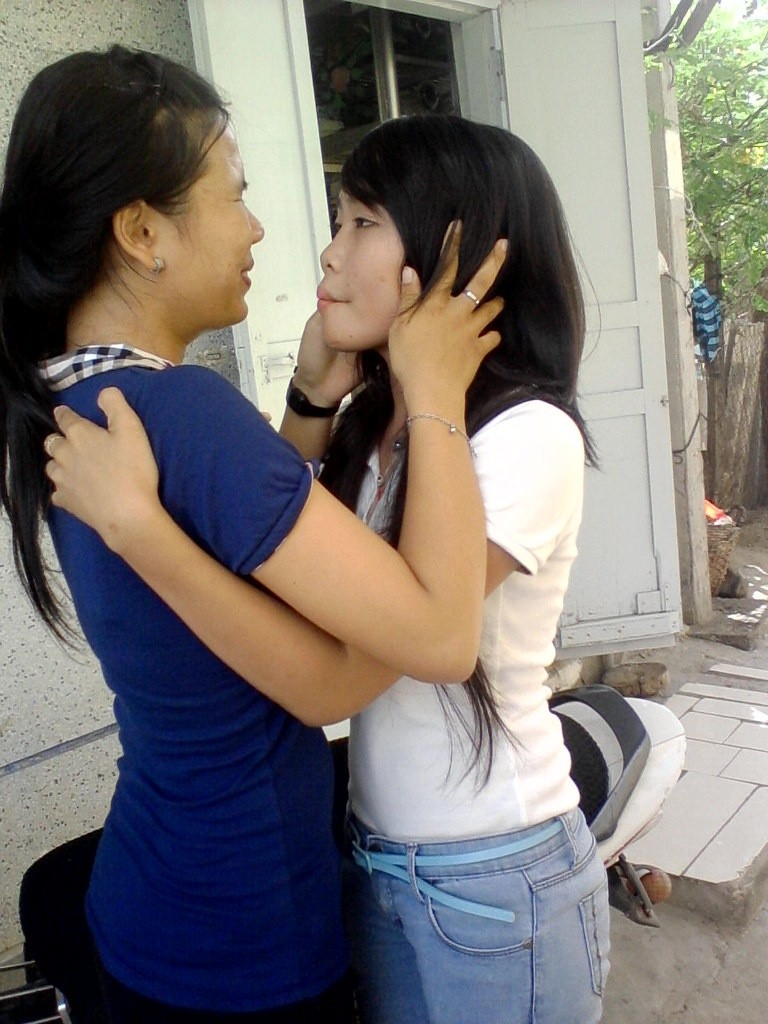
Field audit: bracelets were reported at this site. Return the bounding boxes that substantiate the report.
[404,411,479,463]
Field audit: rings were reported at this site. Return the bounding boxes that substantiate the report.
[465,286,480,307]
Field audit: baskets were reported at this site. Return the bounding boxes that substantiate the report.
[707,505,745,596]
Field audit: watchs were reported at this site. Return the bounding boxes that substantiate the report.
[284,365,341,418]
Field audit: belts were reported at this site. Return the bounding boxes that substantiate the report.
[352,822,564,922]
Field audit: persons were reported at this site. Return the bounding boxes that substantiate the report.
[44,111,616,1024]
[1,40,513,1024]
